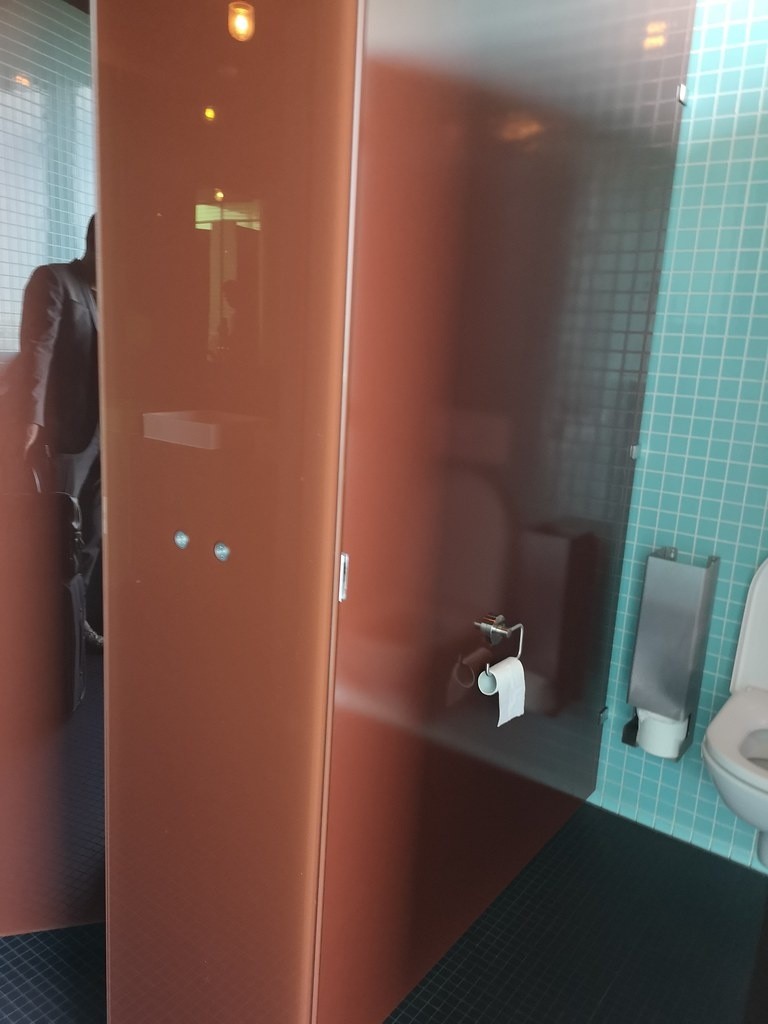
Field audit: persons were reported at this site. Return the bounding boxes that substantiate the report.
[14,212,105,716]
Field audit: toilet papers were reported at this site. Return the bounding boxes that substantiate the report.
[635,708,689,760]
[477,656,526,728]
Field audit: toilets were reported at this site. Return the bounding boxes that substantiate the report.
[701,556,767,877]
